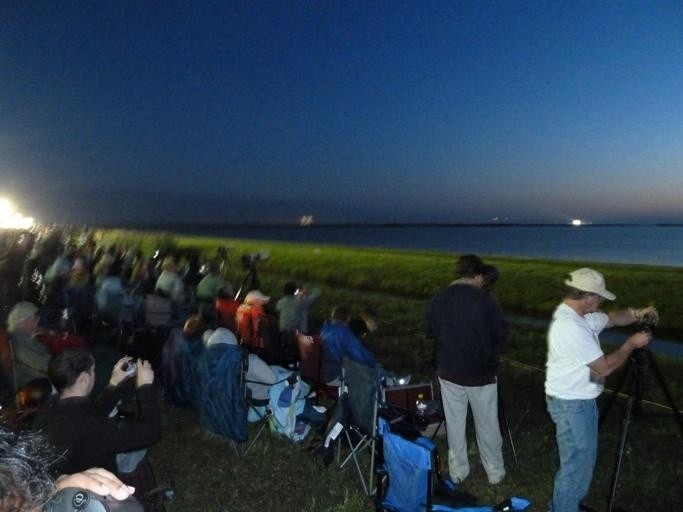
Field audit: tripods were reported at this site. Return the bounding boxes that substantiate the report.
[598,346,682,512]
[232,272,263,301]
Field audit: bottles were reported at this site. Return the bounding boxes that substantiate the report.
[416,394,427,417]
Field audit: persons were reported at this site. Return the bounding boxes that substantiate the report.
[1,224,383,512]
[424,254,514,486]
[544,267,660,511]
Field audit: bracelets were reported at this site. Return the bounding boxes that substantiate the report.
[630,308,640,323]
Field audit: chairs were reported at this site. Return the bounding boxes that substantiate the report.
[238,355,316,456]
[336,355,434,498]
[376,432,493,512]
[0,338,48,407]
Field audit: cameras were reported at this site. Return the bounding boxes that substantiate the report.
[123,361,139,374]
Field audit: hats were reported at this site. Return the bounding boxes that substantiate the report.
[244,291,271,305]
[563,268,616,300]
